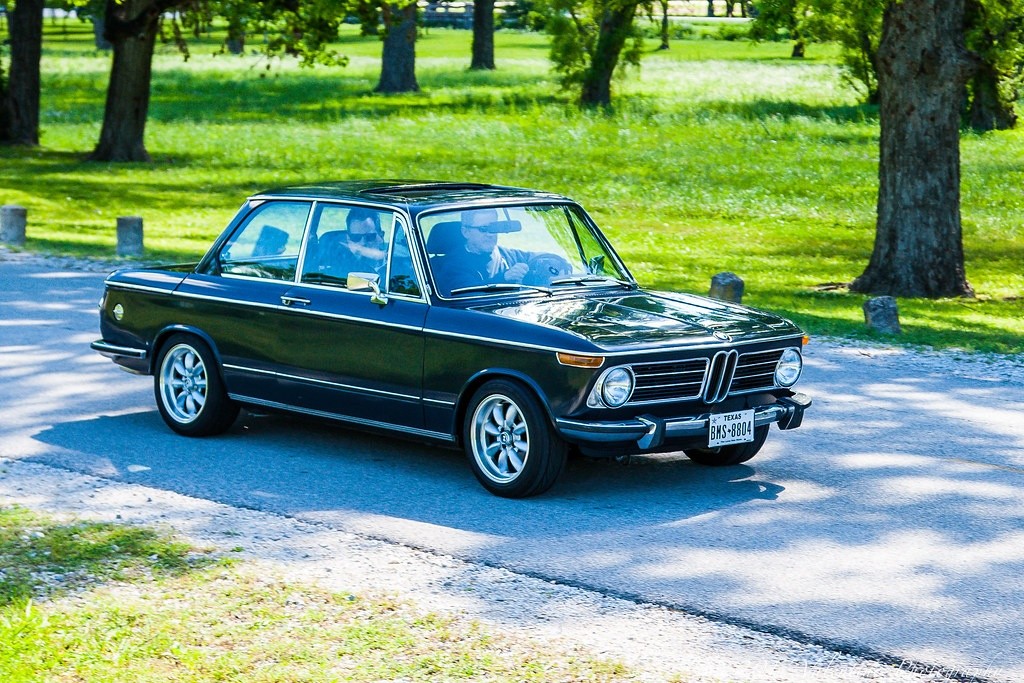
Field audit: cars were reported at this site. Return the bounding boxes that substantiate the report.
[89,177,813,501]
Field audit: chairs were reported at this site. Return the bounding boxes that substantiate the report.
[318,230,350,273]
[427,221,463,272]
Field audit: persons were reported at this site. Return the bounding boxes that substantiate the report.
[439,208,572,294]
[329,208,416,295]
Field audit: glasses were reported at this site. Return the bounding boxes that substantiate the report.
[347,231,379,243]
[465,224,488,233]
